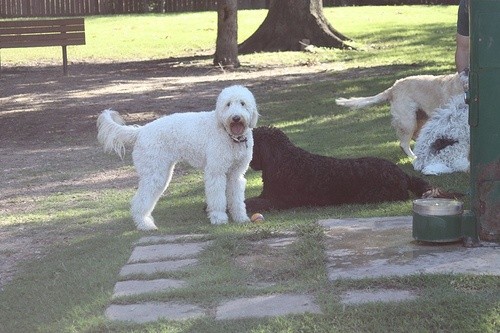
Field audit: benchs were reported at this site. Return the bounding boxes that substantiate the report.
[0,18,86,76]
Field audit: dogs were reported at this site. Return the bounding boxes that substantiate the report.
[334,66,469,158]
[203,125,468,213]
[96,84,260,232]
[408,93,470,176]
